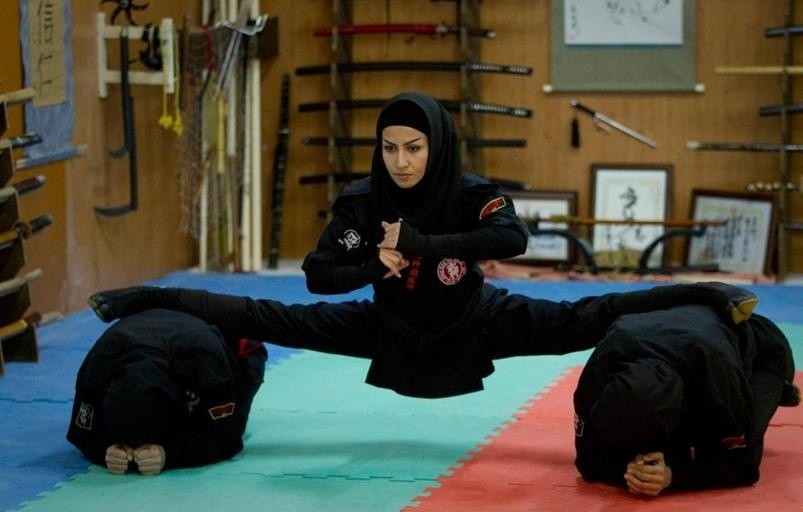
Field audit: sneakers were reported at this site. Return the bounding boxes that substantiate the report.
[87,286,147,324]
[710,282,758,325]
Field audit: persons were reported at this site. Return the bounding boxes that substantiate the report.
[65,308,269,477]
[570,302,801,498]
[87,90,759,398]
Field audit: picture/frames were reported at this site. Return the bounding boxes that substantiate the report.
[497,161,778,278]
